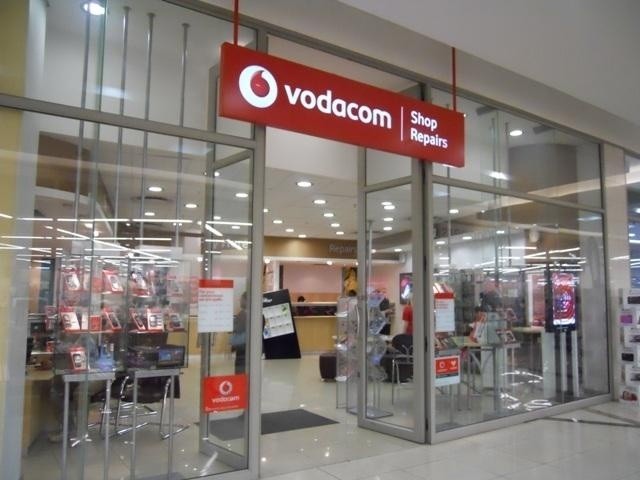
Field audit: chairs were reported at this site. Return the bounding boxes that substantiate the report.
[391,333,414,405]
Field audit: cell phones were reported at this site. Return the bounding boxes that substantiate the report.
[135,315,143,327]
[109,314,118,327]
[73,354,82,367]
[169,280,179,292]
[110,277,120,289]
[150,315,157,327]
[171,315,180,327]
[135,272,144,287]
[66,275,77,288]
[63,314,72,328]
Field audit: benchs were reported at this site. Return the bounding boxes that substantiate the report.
[319,352,413,384]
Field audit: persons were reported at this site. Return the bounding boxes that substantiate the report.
[344,268,357,295]
[401,292,413,336]
[297,297,305,302]
[230,288,265,420]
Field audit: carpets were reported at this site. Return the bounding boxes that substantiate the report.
[194,408,340,442]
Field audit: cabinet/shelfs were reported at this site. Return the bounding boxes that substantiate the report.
[21,253,190,457]
[335,293,393,422]
